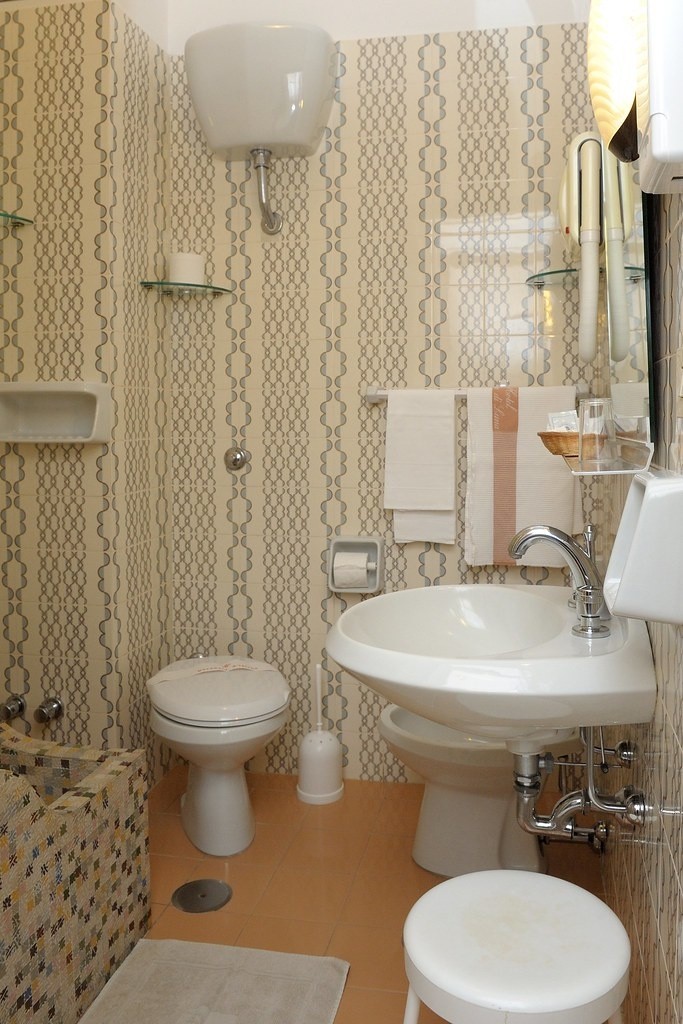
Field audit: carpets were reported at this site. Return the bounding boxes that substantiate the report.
[77,938,350,1024]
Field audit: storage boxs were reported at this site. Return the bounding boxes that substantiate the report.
[537,430,609,457]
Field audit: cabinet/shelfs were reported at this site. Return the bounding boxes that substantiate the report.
[1,379,113,448]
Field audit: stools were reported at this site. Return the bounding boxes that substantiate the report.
[400,866,631,1024]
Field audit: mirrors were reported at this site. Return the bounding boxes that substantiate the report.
[600,141,653,446]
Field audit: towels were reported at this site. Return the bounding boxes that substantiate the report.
[383,383,584,568]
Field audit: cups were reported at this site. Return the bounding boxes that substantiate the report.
[577,398,619,463]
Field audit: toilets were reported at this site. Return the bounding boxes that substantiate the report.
[147,655,292,859]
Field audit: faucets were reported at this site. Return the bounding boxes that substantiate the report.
[507,525,615,621]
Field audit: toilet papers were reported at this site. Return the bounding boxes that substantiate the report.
[165,252,205,288]
[332,551,371,590]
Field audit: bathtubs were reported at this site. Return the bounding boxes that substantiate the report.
[0,719,153,1024]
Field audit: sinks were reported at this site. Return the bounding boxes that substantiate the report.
[325,582,659,757]
[377,703,584,881]
